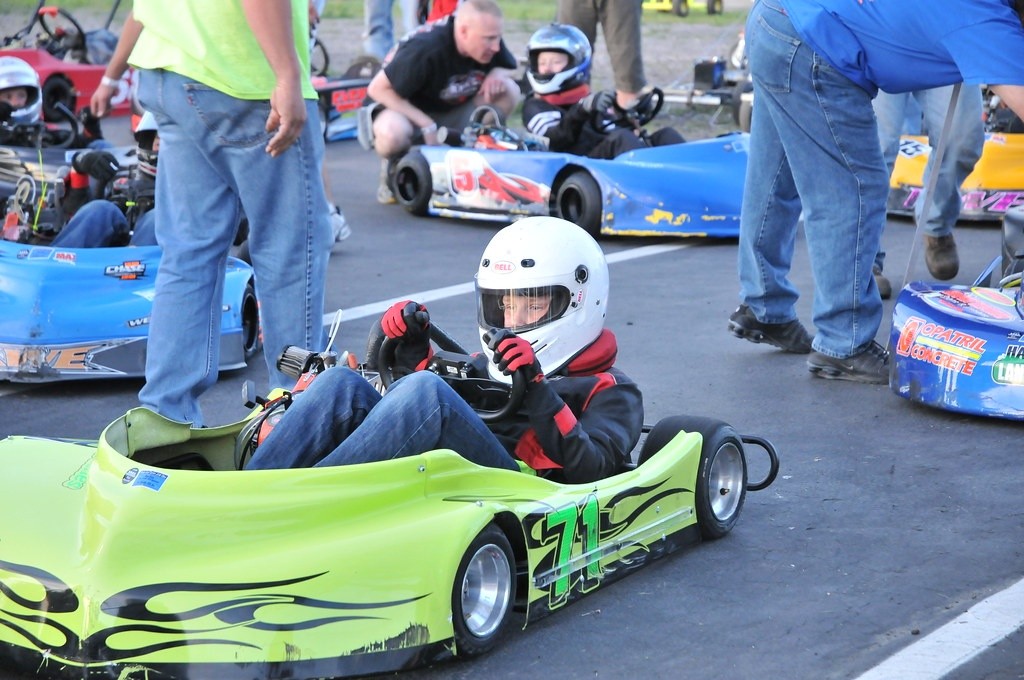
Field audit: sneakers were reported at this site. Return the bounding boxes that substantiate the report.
[925,233,958,281]
[375,186,397,204]
[728,304,814,353]
[806,341,891,386]
[328,203,351,243]
[870,268,892,298]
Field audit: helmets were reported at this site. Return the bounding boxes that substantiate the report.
[521,24,592,95]
[134,111,158,176]
[0,56,42,126]
[475,216,609,386]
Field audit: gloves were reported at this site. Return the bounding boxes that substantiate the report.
[488,330,548,385]
[576,90,616,116]
[380,300,430,353]
[0,101,17,125]
[72,152,119,180]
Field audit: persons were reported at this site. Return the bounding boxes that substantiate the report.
[118,0,337,429]
[245,216,644,485]
[729,0,1024,385]
[0,0,985,297]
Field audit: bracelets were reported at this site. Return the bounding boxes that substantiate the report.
[421,123,438,133]
[101,77,120,89]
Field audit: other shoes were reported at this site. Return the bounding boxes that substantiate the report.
[624,93,653,120]
